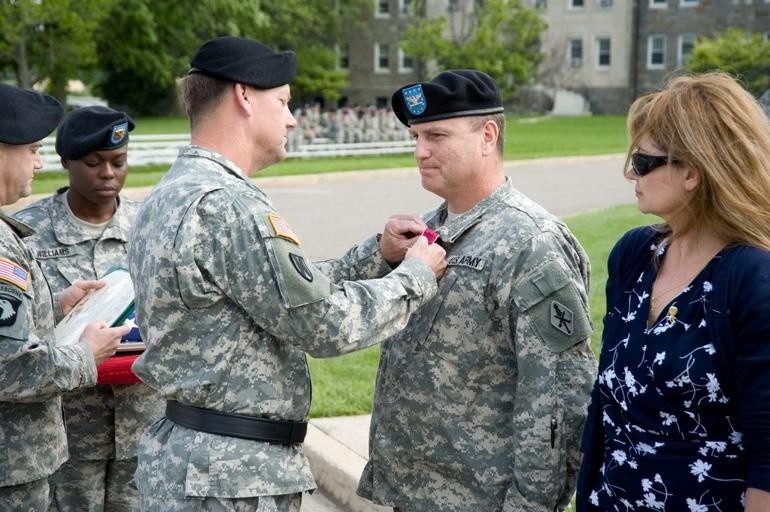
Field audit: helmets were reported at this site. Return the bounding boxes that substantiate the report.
[1,80,64,146]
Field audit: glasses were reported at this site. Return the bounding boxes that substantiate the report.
[629,149,684,178]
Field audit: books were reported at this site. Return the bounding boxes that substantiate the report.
[53,265,137,348]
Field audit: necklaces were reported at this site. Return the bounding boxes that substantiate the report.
[645,259,696,316]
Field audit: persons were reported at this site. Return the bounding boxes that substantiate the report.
[352,69,597,511]
[8,105,148,511]
[572,66,769,510]
[127,35,449,512]
[0,79,135,509]
[285,101,413,154]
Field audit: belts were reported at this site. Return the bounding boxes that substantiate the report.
[158,397,309,449]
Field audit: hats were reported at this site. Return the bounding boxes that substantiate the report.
[185,35,300,88]
[391,67,505,129]
[55,105,136,159]
